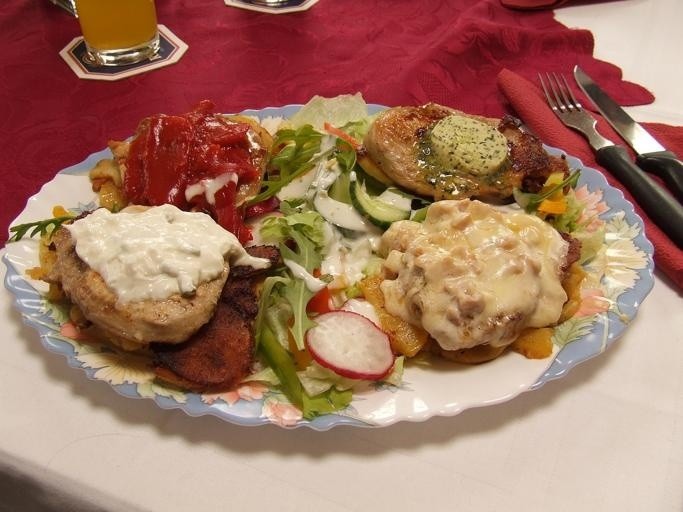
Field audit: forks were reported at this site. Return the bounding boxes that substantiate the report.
[535,71,681,256]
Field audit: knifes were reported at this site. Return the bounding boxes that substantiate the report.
[573,62,682,209]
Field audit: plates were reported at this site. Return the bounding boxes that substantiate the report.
[1,103,656,435]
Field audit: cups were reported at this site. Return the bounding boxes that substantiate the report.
[72,0,163,66]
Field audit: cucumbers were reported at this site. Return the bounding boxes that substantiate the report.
[349,178,413,231]
